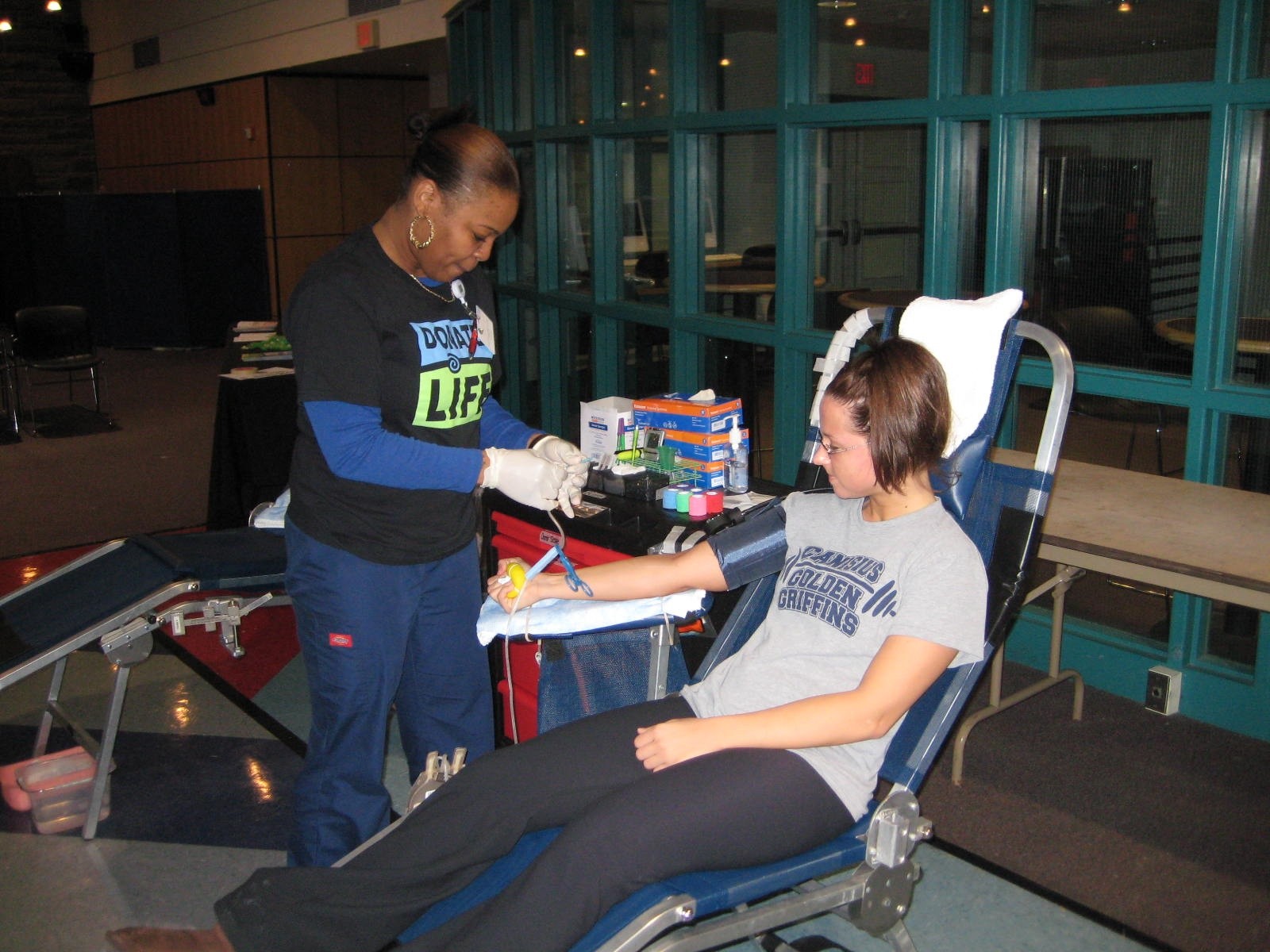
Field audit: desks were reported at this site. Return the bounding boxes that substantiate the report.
[206,322,299,533]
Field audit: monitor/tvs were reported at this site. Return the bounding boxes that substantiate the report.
[624,200,650,258]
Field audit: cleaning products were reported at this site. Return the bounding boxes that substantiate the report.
[722,413,749,494]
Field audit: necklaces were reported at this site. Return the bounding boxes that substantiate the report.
[410,274,456,302]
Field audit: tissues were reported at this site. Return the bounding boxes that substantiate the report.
[632,388,744,434]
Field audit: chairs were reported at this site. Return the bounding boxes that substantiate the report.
[4,305,106,441]
[329,288,1074,952]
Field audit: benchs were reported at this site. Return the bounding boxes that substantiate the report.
[952,449,1270,785]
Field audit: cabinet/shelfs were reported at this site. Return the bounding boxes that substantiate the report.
[491,479,792,746]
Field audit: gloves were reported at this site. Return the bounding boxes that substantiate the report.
[480,446,566,512]
[533,434,589,520]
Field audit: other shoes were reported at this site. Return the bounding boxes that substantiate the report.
[106,921,237,952]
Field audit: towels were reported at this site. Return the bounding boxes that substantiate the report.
[898,285,1025,459]
[475,588,708,649]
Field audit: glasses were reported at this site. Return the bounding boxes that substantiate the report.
[815,437,872,455]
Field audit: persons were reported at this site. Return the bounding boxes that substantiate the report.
[103,338,989,952]
[284,119,593,870]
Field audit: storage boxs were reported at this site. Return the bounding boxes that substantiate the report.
[16,752,116,834]
[580,389,749,501]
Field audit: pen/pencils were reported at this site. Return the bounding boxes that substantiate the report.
[632,425,639,466]
[617,418,625,450]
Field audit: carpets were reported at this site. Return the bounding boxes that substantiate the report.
[916,662,1270,952]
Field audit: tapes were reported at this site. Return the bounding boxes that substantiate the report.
[231,367,258,375]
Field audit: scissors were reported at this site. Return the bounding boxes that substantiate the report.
[554,544,592,597]
